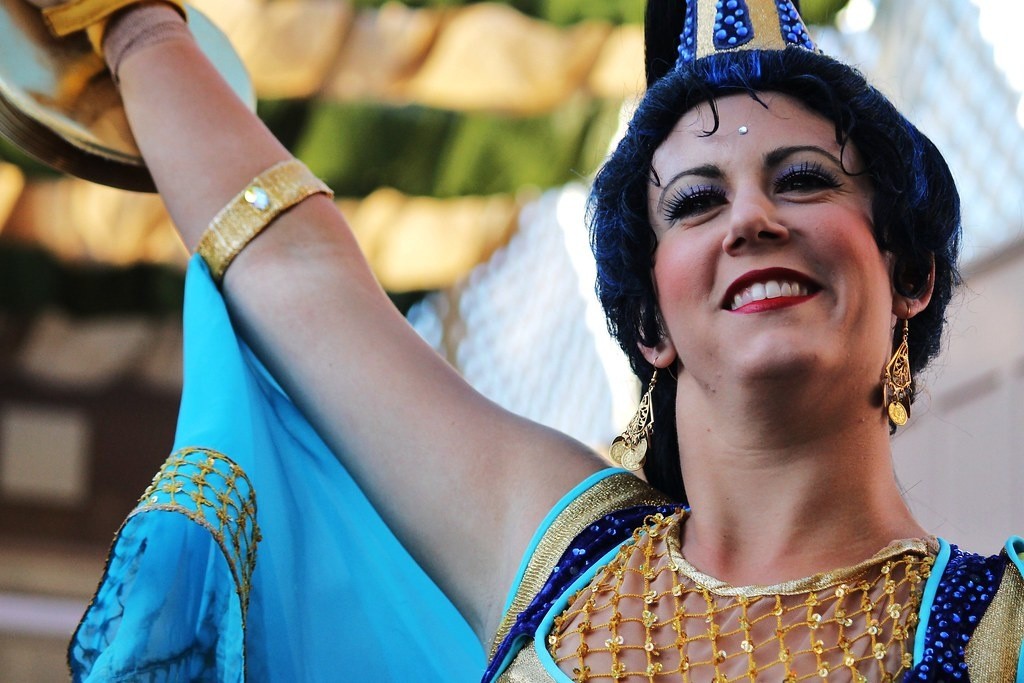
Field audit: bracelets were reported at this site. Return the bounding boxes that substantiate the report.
[98,7,194,84]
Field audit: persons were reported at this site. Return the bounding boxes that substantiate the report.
[26,0,1024,682]
